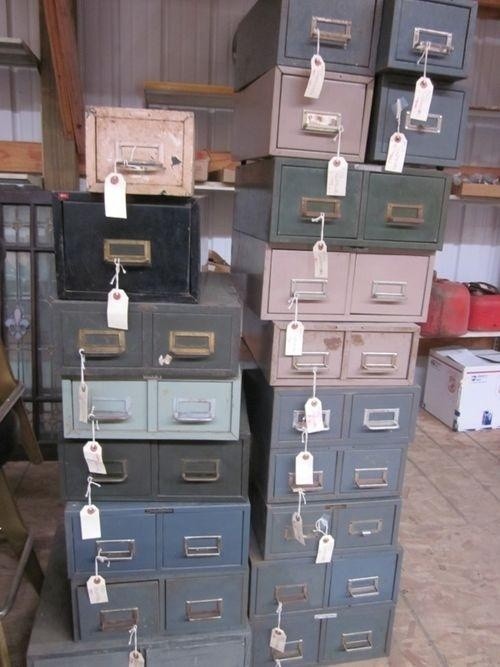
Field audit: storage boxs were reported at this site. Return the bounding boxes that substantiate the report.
[85,104,194,197]
[25,271,421,661]
[52,189,200,302]
[230,5,477,322]
[419,344,500,433]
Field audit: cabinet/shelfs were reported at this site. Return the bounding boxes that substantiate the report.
[140,79,236,193]
